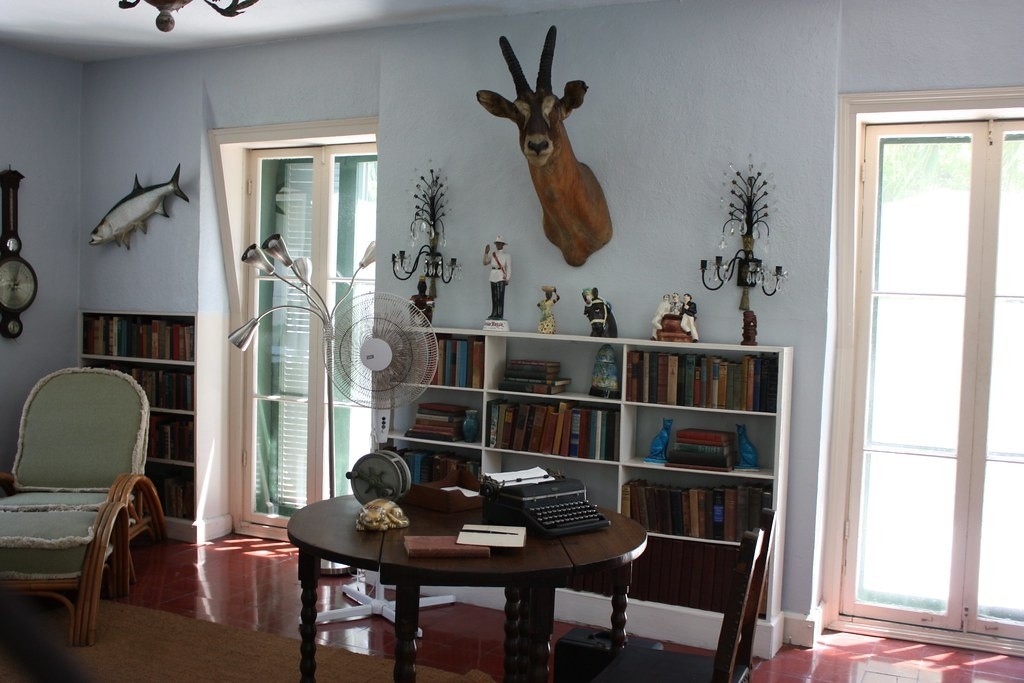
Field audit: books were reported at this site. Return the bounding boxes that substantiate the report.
[497,360,572,395]
[621,480,772,542]
[382,446,481,486]
[151,475,194,519]
[485,398,620,461]
[625,347,779,413]
[404,536,490,558]
[147,414,194,462]
[399,333,483,388]
[404,403,468,442]
[84,314,195,361]
[108,364,194,410]
[562,536,741,612]
[665,428,738,471]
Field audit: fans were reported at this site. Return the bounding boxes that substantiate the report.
[296,285,459,637]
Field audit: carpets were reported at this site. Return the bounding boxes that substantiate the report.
[30,598,496,683]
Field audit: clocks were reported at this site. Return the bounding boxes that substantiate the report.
[0,161,39,340]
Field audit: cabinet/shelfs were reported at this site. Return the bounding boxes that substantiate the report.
[372,320,796,663]
[75,310,198,539]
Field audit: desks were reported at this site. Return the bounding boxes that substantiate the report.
[284,479,650,683]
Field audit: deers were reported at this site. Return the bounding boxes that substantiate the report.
[475,23,615,269]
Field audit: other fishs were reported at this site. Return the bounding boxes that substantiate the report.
[88,162,190,251]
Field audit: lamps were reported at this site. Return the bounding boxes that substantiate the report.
[696,137,791,312]
[227,232,375,578]
[388,152,467,302]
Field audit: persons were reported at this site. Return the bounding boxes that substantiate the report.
[537,286,560,333]
[407,276,437,328]
[483,235,512,320]
[648,293,700,342]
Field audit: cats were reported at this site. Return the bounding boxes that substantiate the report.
[355,498,411,532]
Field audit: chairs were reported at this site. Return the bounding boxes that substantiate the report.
[0,365,170,649]
[580,507,780,683]
